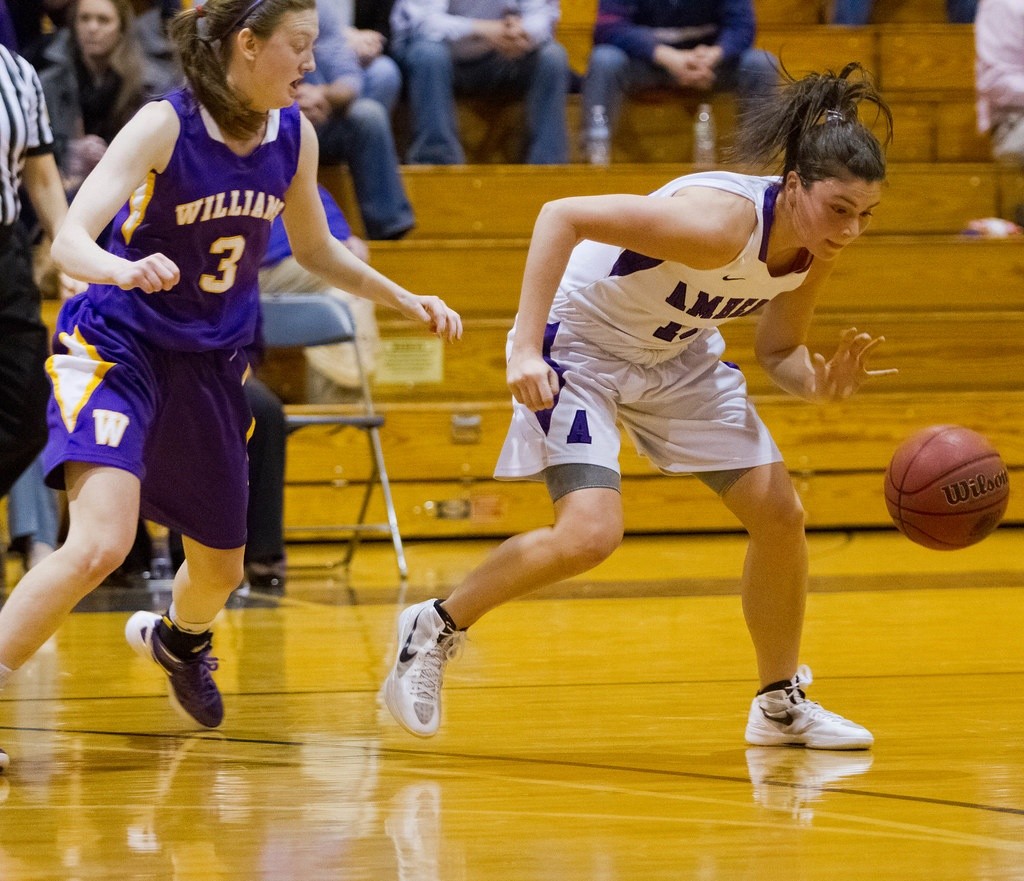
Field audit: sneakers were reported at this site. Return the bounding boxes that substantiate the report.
[0,749,11,776]
[746,665,874,750]
[125,609,225,727]
[387,599,476,739]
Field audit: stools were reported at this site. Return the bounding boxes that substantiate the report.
[256,294,409,581]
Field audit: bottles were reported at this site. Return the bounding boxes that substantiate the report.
[584,106,611,165]
[692,104,718,162]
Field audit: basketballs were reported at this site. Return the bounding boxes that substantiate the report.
[881,426,1011,551]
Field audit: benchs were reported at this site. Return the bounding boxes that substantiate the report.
[451,64,712,163]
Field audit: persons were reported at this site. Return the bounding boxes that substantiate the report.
[36,0,415,241]
[0,44,70,497]
[579,0,779,165]
[388,0,569,165]
[972,0,1024,156]
[386,59,893,750]
[0,0,462,774]
